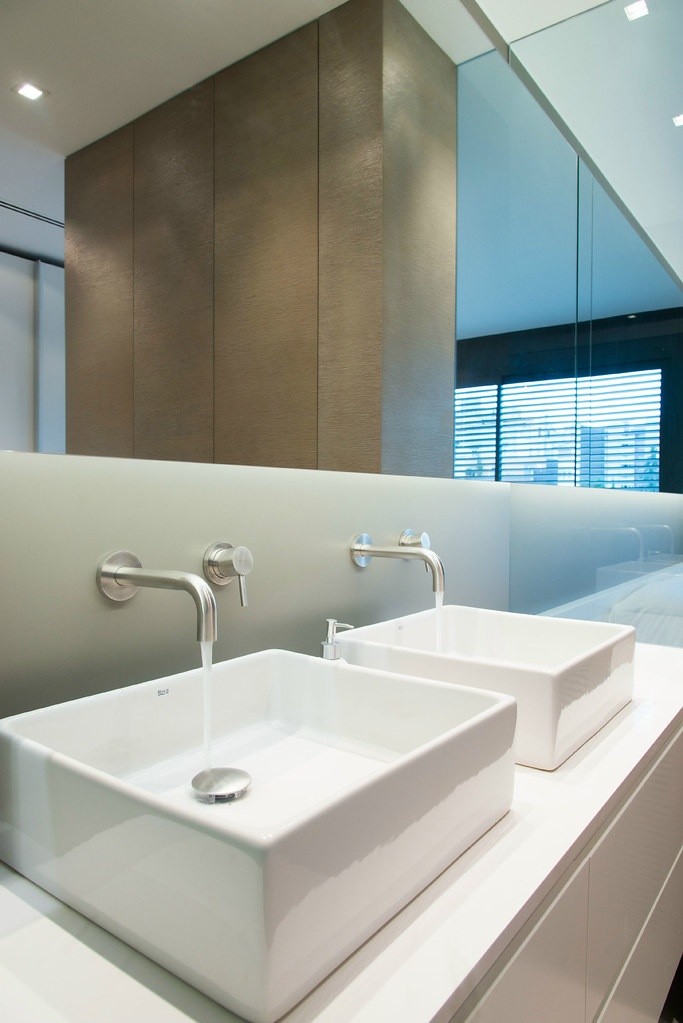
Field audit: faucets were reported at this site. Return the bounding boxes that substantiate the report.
[350,531,447,593]
[644,549,662,562]
[96,551,218,642]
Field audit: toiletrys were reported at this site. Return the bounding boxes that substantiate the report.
[320,619,354,664]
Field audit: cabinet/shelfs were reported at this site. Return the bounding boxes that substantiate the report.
[454,723,683,1023]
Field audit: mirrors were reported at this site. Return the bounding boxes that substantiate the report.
[0,0,683,500]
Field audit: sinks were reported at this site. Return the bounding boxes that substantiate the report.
[1,649,517,1023]
[325,604,636,771]
[595,552,683,617]
